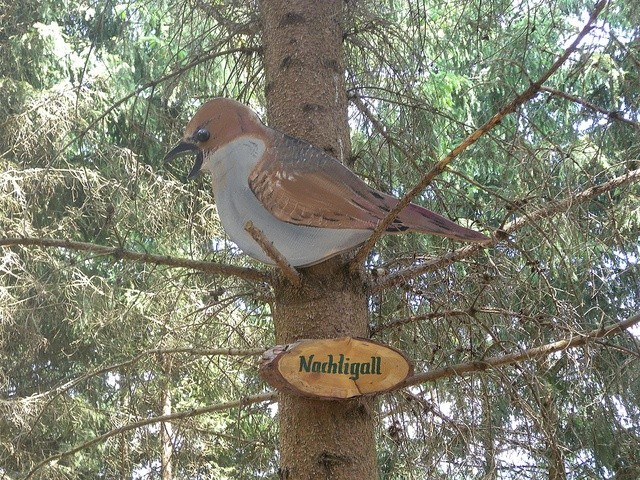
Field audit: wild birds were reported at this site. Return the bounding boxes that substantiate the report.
[164,97,491,269]
